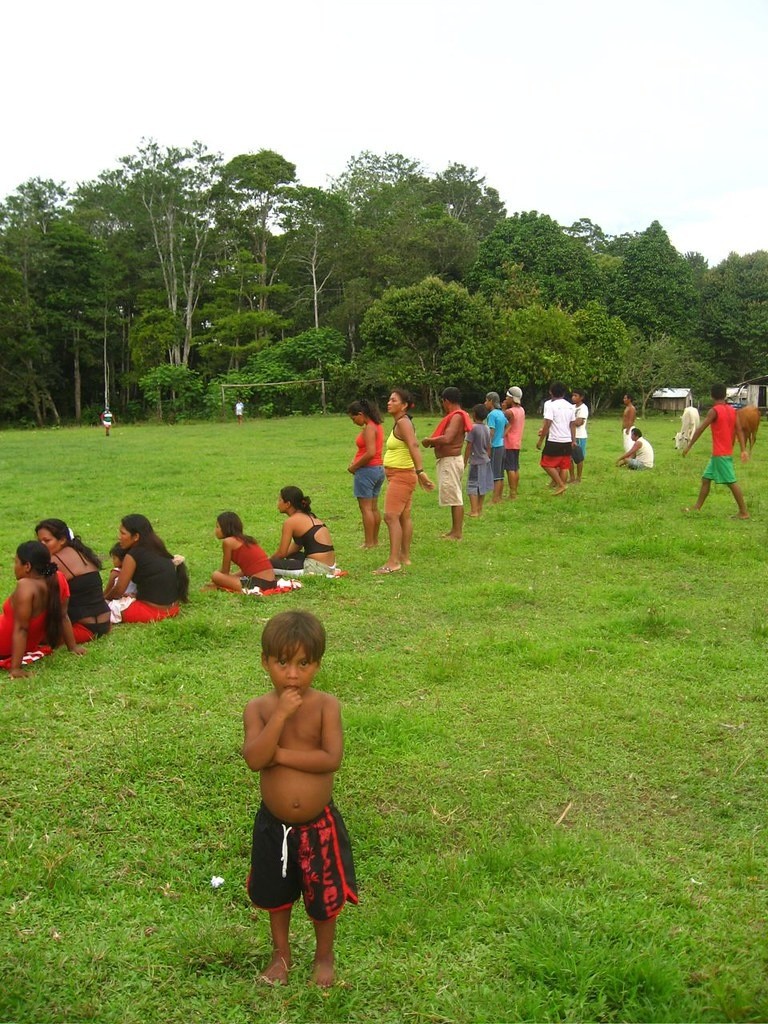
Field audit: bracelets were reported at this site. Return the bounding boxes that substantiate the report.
[416,469,423,474]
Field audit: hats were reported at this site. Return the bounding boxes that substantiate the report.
[487,392,502,409]
[506,386,523,404]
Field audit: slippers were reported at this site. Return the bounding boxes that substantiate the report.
[371,566,402,576]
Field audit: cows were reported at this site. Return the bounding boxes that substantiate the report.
[735,404,759,458]
[672,406,701,448]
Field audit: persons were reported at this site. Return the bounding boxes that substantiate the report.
[615,428,654,471]
[464,404,494,516]
[104,514,179,624]
[422,387,474,541]
[537,382,577,496]
[347,398,384,550]
[269,485,335,575]
[236,400,244,424]
[204,512,276,593]
[622,394,636,466]
[567,387,589,484]
[0,541,87,680]
[35,519,111,644]
[681,384,752,520]
[503,385,526,501]
[377,389,436,575]
[101,407,115,436]
[242,610,361,988]
[485,391,512,506]
